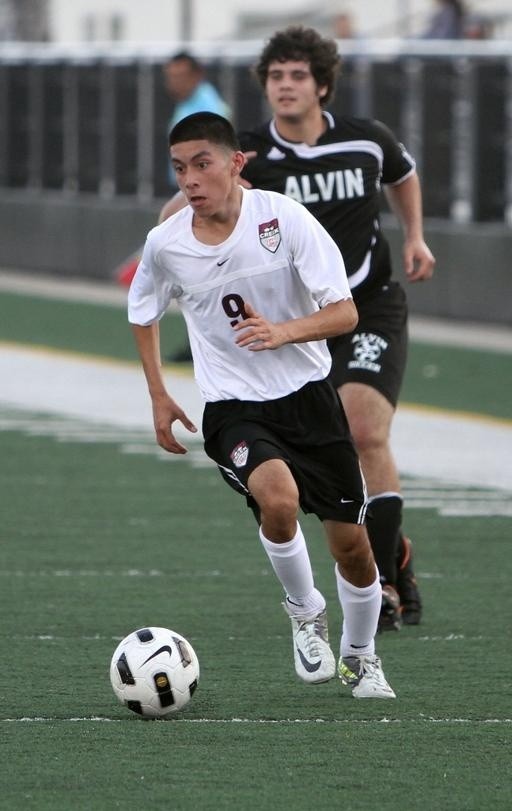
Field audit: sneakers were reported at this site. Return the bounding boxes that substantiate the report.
[337,655,397,698]
[378,535,421,632]
[281,602,336,684]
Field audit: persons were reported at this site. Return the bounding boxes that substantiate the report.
[128,112,396,698]
[161,48,241,362]
[418,1,465,42]
[157,23,437,631]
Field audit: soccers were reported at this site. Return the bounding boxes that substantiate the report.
[110,628,199,717]
